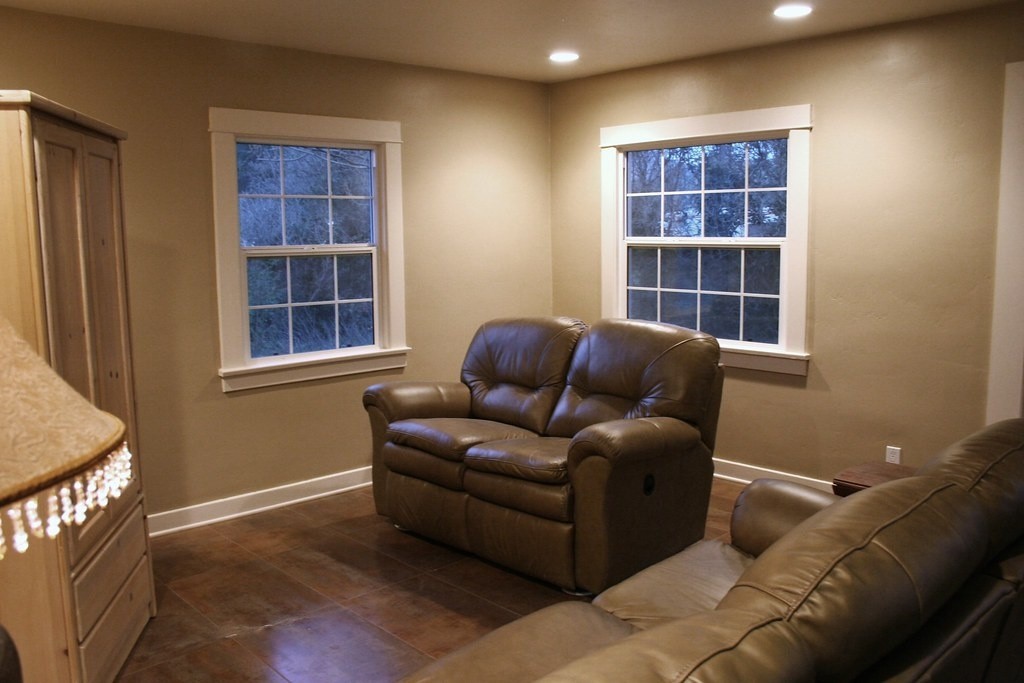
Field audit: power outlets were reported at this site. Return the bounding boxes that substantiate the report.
[885,445,902,466]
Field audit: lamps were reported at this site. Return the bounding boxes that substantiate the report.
[0,313,134,683]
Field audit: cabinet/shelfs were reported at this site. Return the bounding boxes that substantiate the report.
[0,88,160,683]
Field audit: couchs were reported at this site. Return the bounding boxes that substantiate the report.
[416,416,1024,683]
[365,315,727,595]
